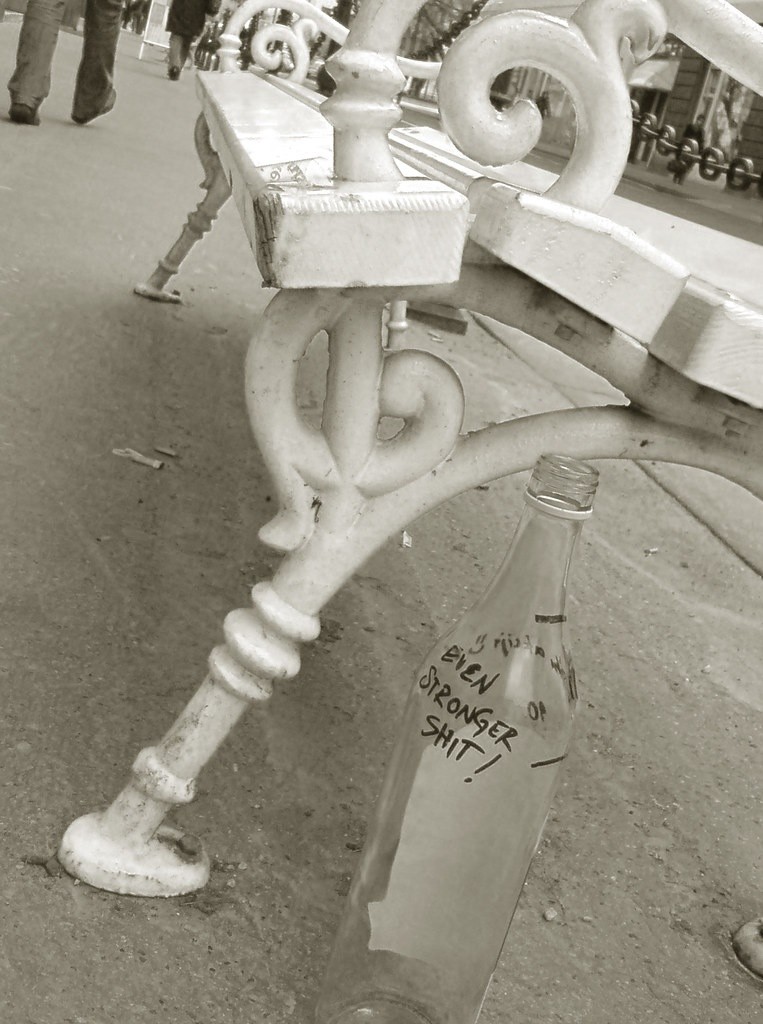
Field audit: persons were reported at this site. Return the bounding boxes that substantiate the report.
[671,113,707,184]
[8,1,123,126]
[164,1,220,80]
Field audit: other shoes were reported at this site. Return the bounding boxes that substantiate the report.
[9,103,41,126]
[169,66,179,80]
[71,90,116,123]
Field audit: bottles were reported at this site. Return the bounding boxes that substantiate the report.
[309,452,599,1023]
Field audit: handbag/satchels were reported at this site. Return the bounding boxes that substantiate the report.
[207,0,221,17]
